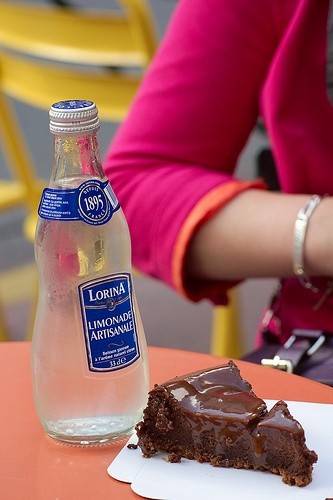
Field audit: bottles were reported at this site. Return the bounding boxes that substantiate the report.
[31,100,150,448]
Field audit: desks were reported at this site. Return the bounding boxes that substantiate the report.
[0,340,333,500]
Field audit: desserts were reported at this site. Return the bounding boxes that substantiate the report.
[127,359,318,488]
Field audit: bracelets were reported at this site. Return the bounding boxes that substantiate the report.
[293,193,332,293]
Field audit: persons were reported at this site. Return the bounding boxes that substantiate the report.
[104,0,333,345]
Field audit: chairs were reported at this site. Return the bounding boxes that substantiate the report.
[0,0,245,357]
